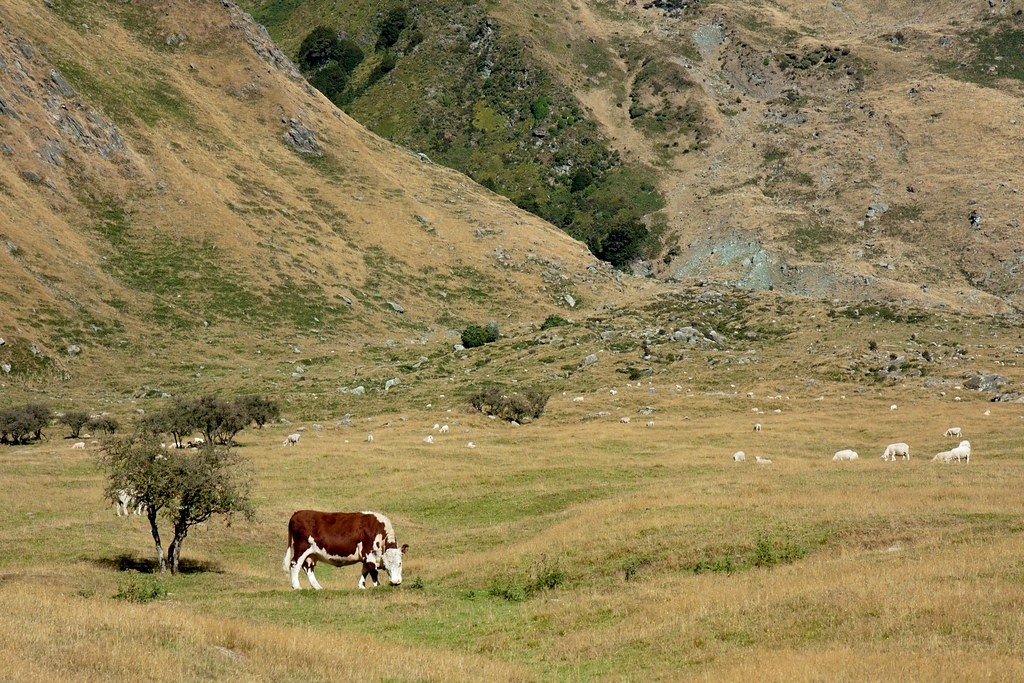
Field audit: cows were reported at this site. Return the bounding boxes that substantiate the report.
[281,509,410,593]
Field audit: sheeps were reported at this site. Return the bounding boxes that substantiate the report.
[71,302,1024,517]
